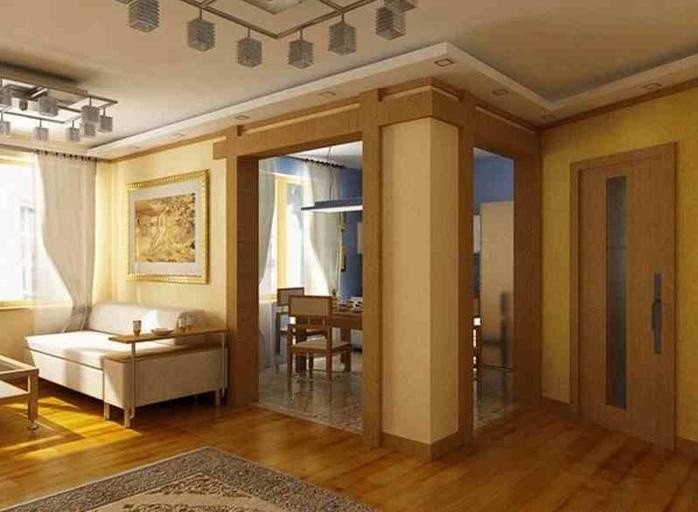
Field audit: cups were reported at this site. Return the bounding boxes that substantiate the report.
[132,320,141,338]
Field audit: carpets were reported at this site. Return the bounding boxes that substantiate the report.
[1,444,382,512]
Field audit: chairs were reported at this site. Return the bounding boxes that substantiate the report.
[276,287,353,381]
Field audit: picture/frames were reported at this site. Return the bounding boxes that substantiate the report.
[125,169,209,285]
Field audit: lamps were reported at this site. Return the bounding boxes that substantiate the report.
[1,70,118,144]
[124,0,422,69]
[300,146,366,214]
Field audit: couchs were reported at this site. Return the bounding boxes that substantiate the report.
[22,301,230,429]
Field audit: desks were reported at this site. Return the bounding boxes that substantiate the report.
[331,303,364,372]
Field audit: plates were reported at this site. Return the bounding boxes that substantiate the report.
[151,330,173,336]
